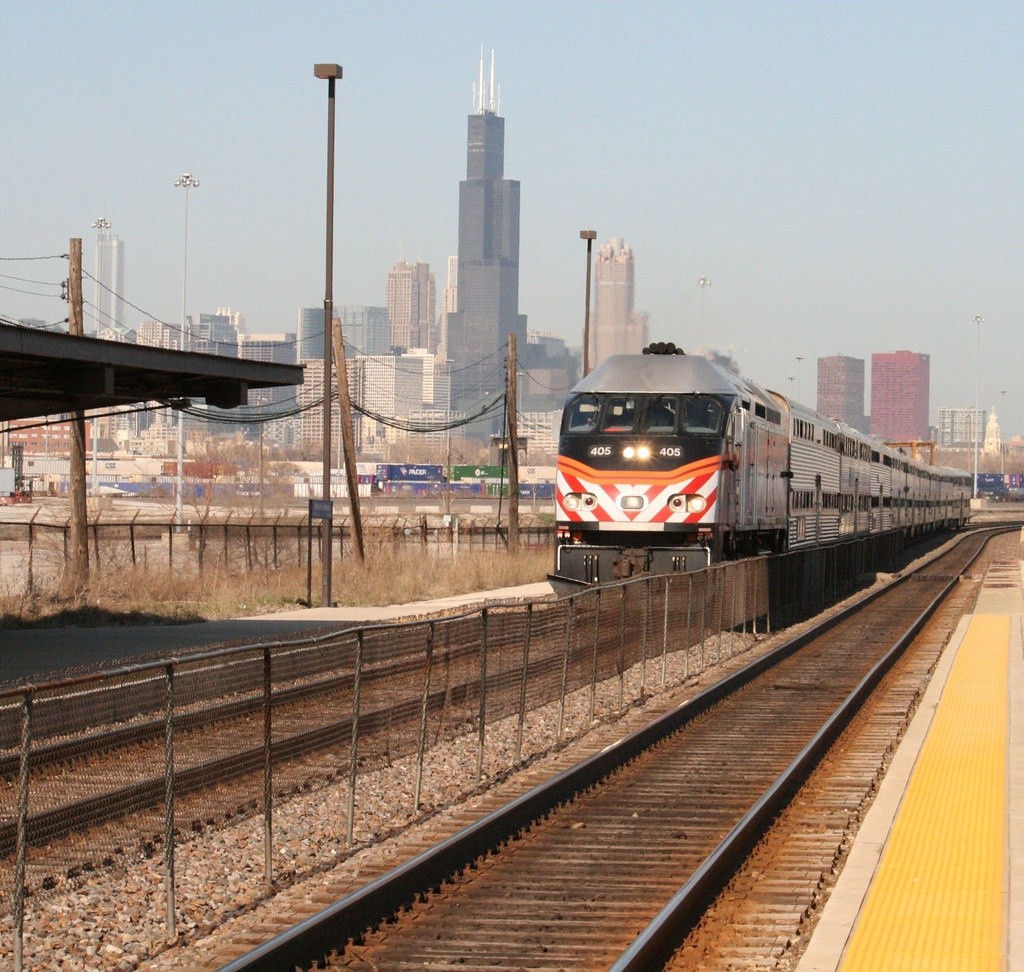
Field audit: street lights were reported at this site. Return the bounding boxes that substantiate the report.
[311,61,346,611]
[580,230,596,378]
[91,217,114,497]
[172,173,203,531]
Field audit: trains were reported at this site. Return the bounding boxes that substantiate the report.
[543,340,974,629]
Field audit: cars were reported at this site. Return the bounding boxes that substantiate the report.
[976,486,1024,503]
